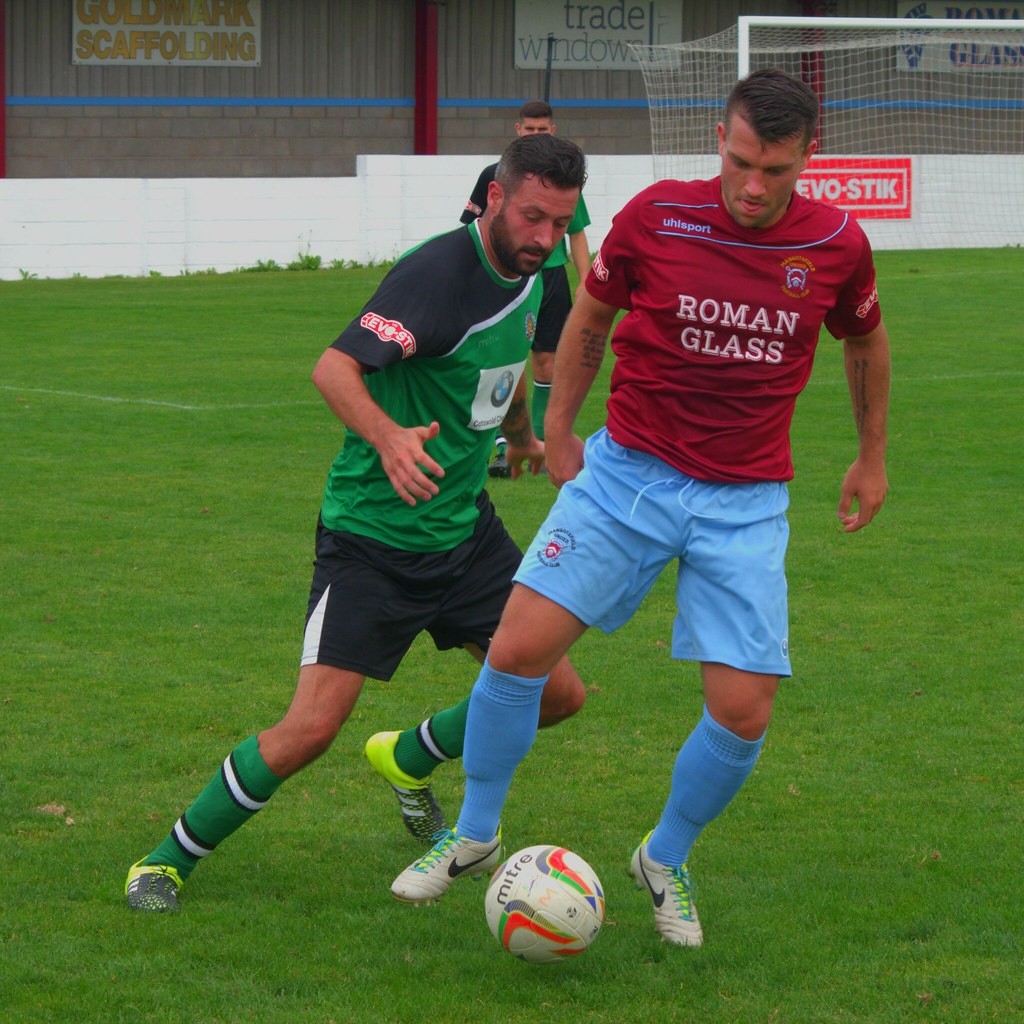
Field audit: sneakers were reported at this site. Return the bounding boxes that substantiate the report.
[527,459,548,473]
[389,821,503,906]
[363,730,447,845]
[630,830,703,947]
[487,445,511,478]
[125,855,183,914]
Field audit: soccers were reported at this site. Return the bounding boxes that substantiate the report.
[484,844,605,964]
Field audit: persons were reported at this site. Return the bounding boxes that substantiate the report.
[124,133,587,907]
[460,99,592,477]
[391,65,890,947]
